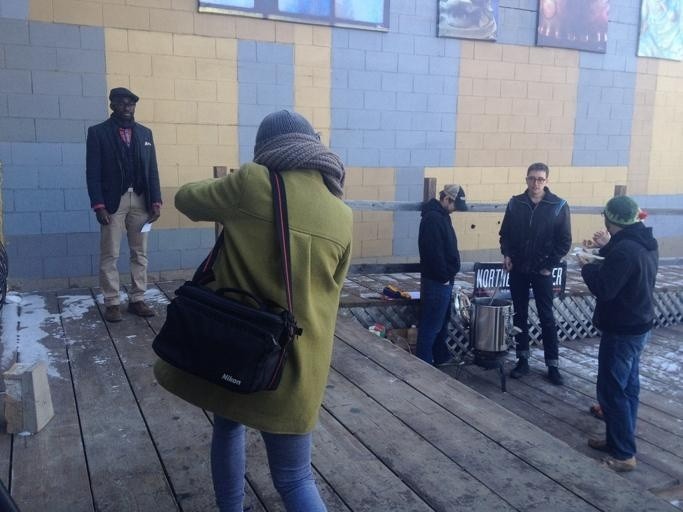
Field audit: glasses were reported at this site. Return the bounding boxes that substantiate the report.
[526,175,550,187]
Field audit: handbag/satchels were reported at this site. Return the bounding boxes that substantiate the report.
[151,167,303,395]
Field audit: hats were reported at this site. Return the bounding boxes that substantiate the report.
[600,197,648,227]
[110,86,142,100]
[442,182,468,213]
[254,110,316,145]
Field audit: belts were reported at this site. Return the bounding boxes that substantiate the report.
[127,187,139,193]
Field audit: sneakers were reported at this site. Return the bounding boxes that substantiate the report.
[127,302,155,316]
[547,367,564,386]
[510,359,529,379]
[105,305,122,322]
[598,455,636,472]
[437,357,465,367]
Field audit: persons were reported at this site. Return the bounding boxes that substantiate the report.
[583,205,648,422]
[87,87,162,322]
[414,183,465,367]
[499,163,572,386]
[577,195,658,472]
[154,109,354,508]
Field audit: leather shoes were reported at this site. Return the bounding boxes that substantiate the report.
[590,401,606,419]
[588,436,611,452]
